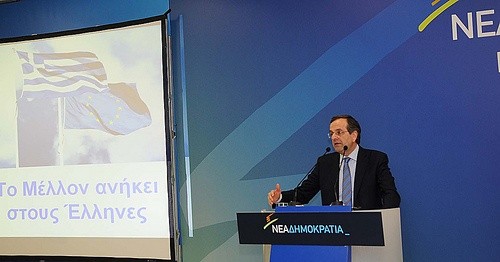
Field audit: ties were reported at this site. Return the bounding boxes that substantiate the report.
[342,158,352,207]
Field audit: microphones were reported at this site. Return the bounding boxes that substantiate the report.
[329,146,349,205]
[287,147,330,207]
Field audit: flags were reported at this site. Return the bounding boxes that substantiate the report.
[17,50,110,97]
[64,79,152,135]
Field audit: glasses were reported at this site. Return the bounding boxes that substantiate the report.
[328,130,353,137]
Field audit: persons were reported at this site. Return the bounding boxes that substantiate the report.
[267,114,401,211]
